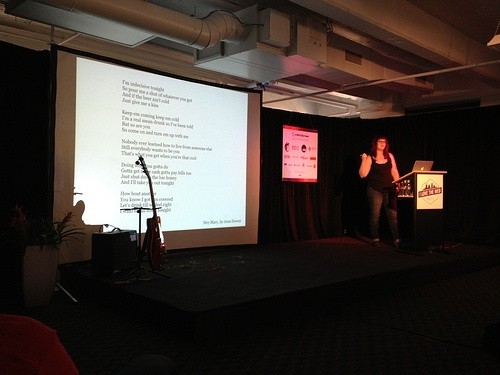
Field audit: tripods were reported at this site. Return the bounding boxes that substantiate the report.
[117,206,171,283]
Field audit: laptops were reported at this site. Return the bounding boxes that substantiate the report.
[412,160,434,171]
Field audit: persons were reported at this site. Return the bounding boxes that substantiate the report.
[359,137,401,247]
[0,313,80,375]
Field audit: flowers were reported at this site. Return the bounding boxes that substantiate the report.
[9,208,90,251]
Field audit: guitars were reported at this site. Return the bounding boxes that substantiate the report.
[135,155,165,272]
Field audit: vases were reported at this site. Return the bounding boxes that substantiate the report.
[19,245,59,305]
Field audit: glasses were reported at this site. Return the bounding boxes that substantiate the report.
[377,141,387,144]
